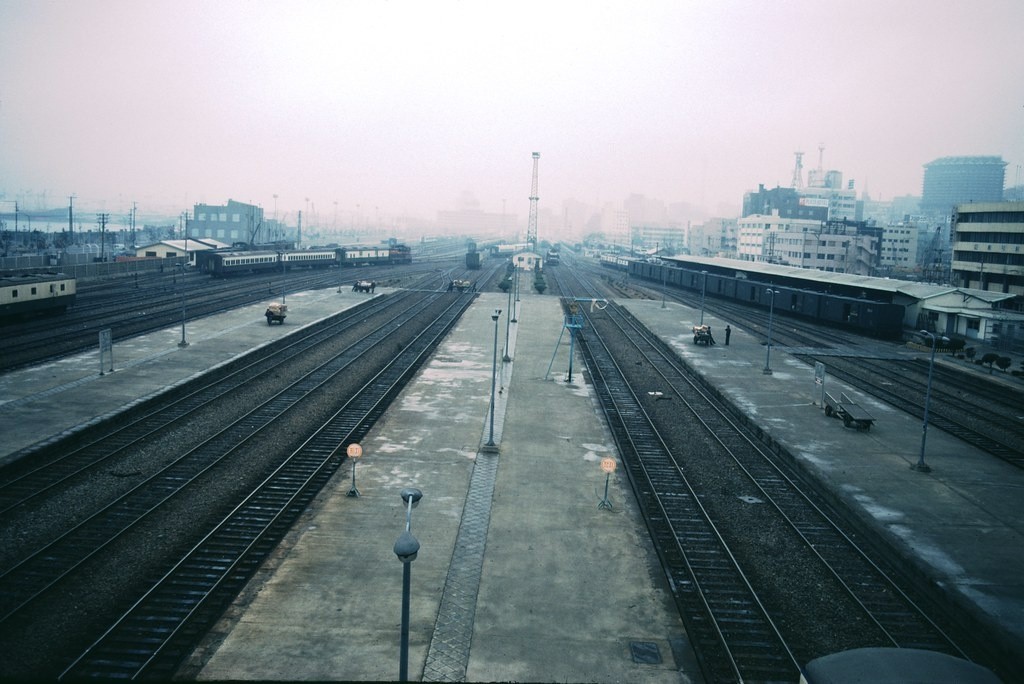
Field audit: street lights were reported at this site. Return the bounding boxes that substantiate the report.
[762,289,780,375]
[701,271,708,325]
[281,252,289,304]
[482,308,503,452]
[910,331,951,472]
[393,488,424,681]
[176,262,191,347]
[503,277,513,363]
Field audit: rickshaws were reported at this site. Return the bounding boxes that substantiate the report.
[265,314,287,326]
[453,285,471,291]
[692,330,712,344]
[356,286,373,291]
[840,403,877,432]
[824,391,854,420]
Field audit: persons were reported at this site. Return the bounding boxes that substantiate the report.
[351,281,360,293]
[265,309,272,326]
[370,280,376,293]
[725,325,731,346]
[707,326,716,345]
[471,282,478,293]
[447,281,454,293]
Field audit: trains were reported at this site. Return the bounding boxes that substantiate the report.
[547,249,560,265]
[601,254,905,339]
[209,245,413,277]
[467,244,534,269]
[801,648,999,684]
